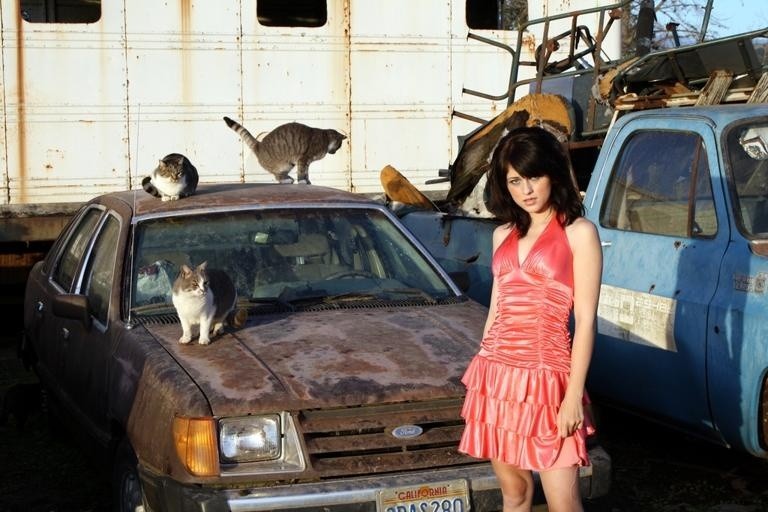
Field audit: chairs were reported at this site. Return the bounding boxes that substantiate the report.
[252,228,365,299]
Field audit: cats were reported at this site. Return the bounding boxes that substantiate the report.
[222,116,348,185]
[142,153,199,201]
[171,260,248,344]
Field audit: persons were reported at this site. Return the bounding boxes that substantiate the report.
[457,126,603,512]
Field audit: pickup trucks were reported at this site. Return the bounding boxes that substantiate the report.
[380,98,768,467]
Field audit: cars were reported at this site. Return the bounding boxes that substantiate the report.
[19,181,620,512]
